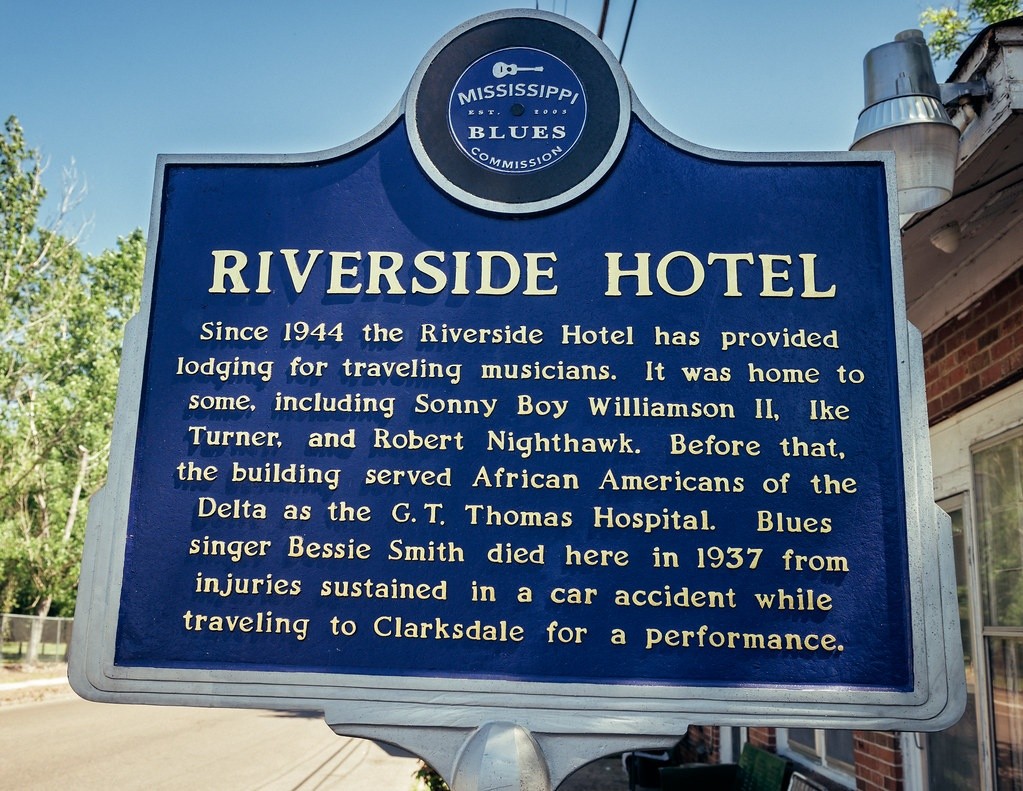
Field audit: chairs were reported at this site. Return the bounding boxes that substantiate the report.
[622,733,829,791]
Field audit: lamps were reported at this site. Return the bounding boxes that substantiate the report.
[847,35,990,218]
